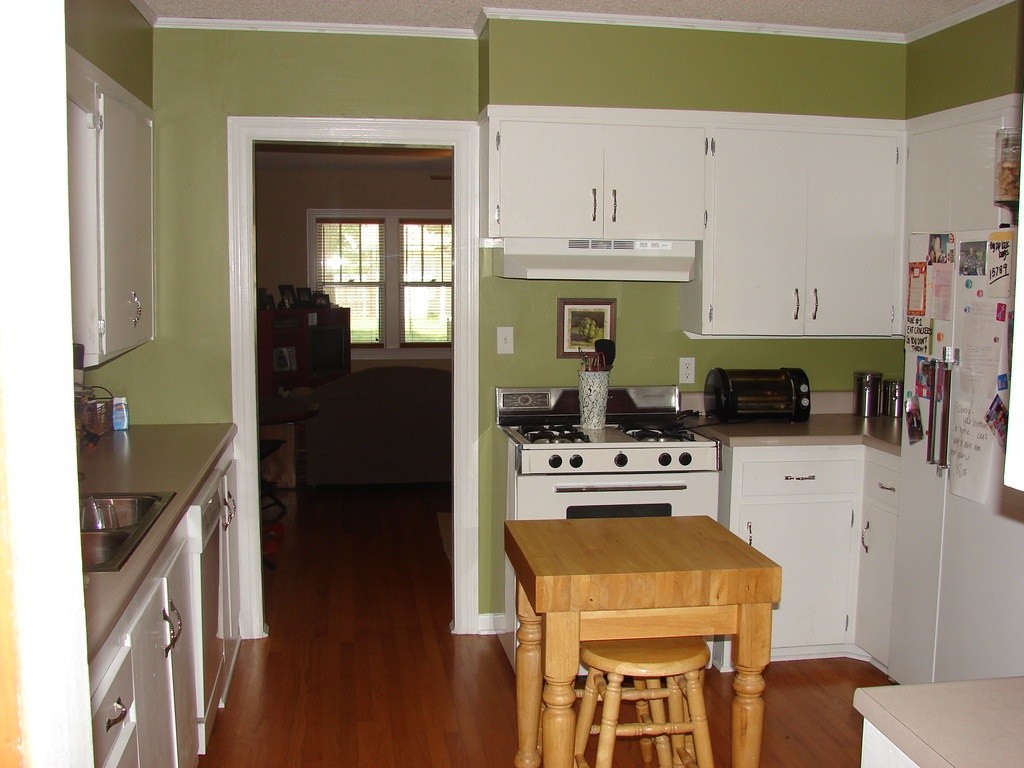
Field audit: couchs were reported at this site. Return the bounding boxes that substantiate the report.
[293,364,451,502]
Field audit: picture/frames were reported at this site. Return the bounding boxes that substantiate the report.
[295,287,313,307]
[556,298,617,359]
[278,285,296,309]
[312,294,330,308]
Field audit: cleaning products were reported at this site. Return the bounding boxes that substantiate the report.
[113,397,129,430]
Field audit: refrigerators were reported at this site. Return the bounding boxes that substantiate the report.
[887,229,1024,685]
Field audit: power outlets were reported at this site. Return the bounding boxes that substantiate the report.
[679,356,695,384]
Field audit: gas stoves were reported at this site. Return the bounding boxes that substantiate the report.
[493,385,715,443]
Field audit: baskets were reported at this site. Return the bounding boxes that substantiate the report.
[75,385,114,437]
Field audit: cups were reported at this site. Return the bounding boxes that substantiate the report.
[79,500,120,530]
[577,371,610,430]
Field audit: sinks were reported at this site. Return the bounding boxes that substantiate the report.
[81,492,177,531]
[83,531,148,572]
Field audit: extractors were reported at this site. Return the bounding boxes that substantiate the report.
[491,237,696,282]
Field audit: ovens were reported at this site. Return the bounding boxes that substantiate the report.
[505,437,722,676]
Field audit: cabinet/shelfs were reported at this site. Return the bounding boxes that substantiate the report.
[258,307,351,397]
[117,516,199,768]
[852,444,900,675]
[89,624,139,768]
[184,440,242,754]
[899,93,1022,339]
[713,444,862,673]
[67,78,157,368]
[679,110,905,341]
[477,104,710,242]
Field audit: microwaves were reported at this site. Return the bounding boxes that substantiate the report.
[703,367,811,422]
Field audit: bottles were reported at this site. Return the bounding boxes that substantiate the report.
[854,371,883,417]
[884,379,904,417]
[113,397,129,431]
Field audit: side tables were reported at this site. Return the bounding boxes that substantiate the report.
[259,423,305,489]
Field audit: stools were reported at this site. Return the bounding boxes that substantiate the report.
[573,635,715,768]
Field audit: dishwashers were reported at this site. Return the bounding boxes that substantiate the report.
[189,467,225,756]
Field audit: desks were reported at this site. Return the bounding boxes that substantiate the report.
[503,514,782,768]
[259,439,288,521]
